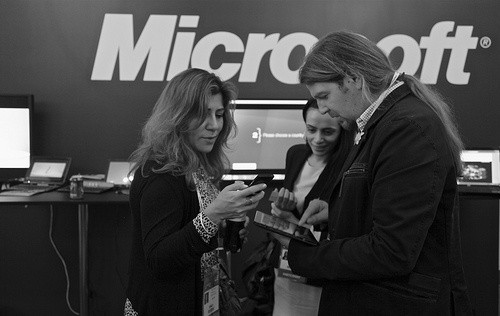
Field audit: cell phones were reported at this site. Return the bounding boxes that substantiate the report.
[248,174,275,187]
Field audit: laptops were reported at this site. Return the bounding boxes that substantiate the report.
[0,155,73,192]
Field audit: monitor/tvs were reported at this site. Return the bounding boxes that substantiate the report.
[221,98,309,180]
[0,94,34,183]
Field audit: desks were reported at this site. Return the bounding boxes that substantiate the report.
[0,178,500,316]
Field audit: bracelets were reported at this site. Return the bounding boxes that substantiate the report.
[200,211,218,236]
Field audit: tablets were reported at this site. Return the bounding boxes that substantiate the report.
[104,158,139,188]
[57,178,114,194]
[254,210,319,247]
[456,149,500,186]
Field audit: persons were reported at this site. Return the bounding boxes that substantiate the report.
[268,93,356,316]
[268,30,464,316]
[117,67,267,316]
